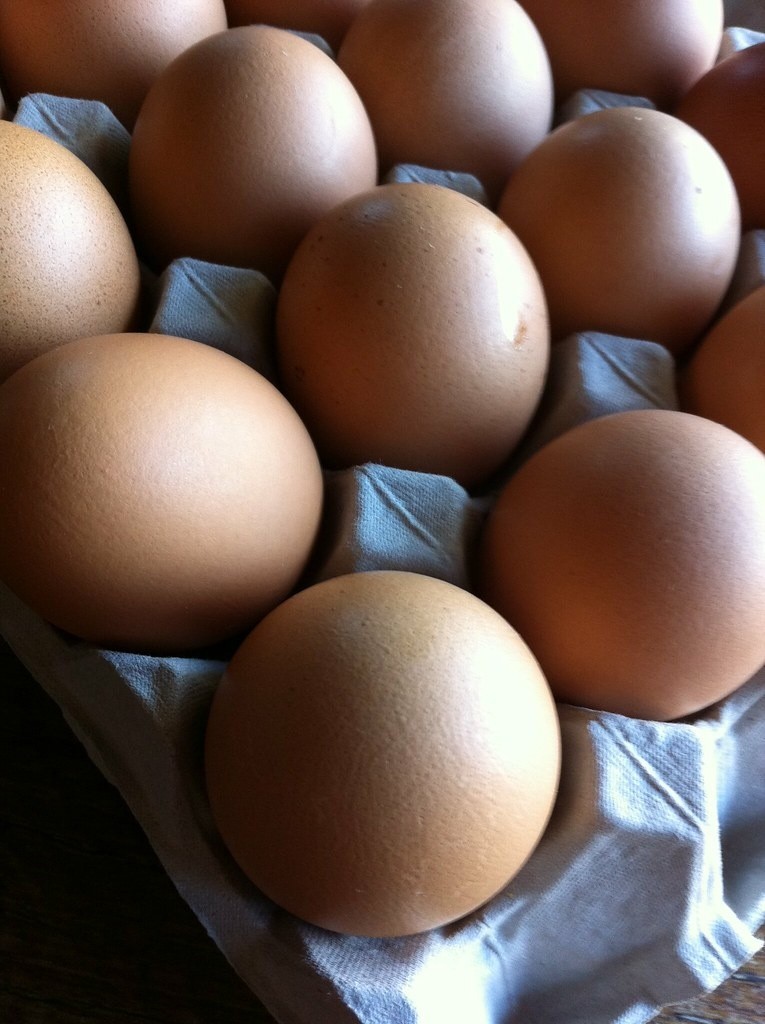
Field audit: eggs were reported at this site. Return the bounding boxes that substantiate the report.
[0,0,765,941]
